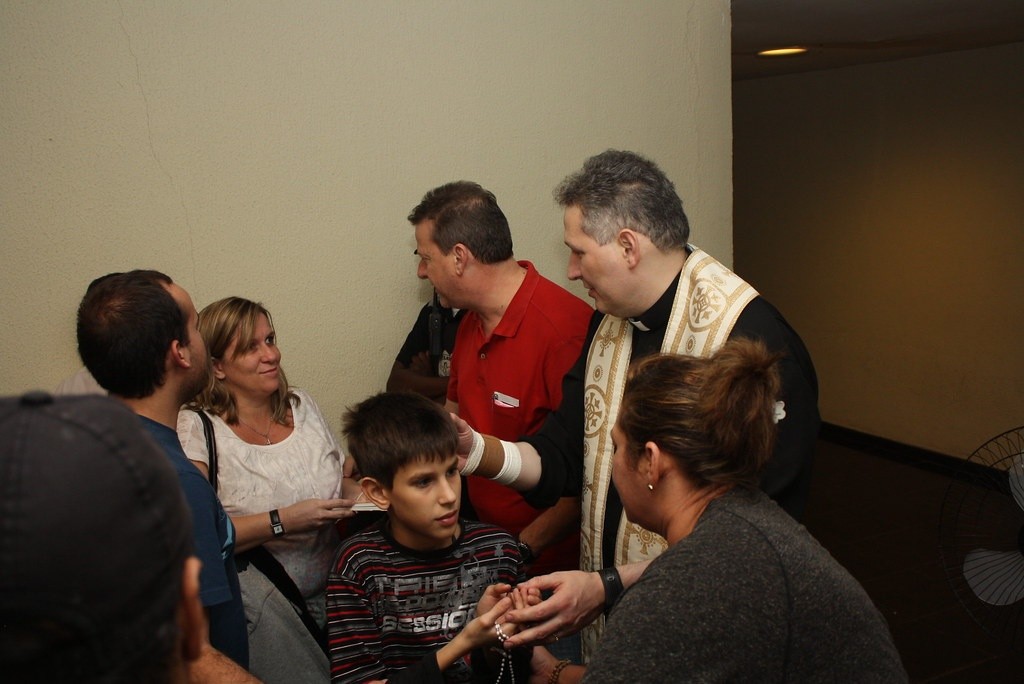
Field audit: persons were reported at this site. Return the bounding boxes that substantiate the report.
[176,296,356,620]
[450,149,820,665]
[387,291,468,411]
[345,183,597,665]
[1,271,265,683]
[507,341,909,684]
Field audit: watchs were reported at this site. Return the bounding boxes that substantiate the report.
[270,509,284,538]
[515,534,536,564]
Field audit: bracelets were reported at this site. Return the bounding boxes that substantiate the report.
[549,660,571,684]
[598,568,624,611]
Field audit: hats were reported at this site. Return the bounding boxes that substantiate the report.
[0,392,193,665]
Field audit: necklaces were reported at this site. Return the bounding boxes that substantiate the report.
[496,624,515,684]
[240,415,272,445]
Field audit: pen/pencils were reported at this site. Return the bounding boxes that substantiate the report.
[494,394,498,400]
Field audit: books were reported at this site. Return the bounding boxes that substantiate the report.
[352,503,387,512]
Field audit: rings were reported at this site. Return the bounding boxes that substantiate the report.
[553,634,560,641]
